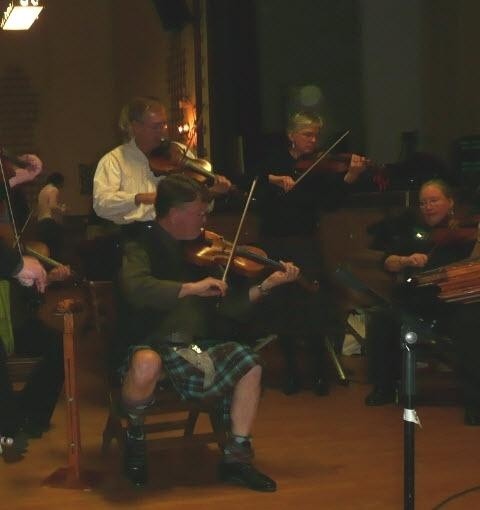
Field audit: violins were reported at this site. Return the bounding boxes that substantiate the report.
[298,148,387,192]
[429,211,480,244]
[184,227,320,296]
[0,145,32,171]
[22,241,96,289]
[149,142,247,200]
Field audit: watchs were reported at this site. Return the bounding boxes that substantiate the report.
[256,280,271,297]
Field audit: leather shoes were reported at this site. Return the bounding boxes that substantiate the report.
[218,461,277,491]
[123,448,147,487]
[365,384,394,406]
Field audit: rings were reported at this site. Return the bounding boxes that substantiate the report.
[354,160,358,162]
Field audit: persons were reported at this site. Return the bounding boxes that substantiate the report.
[93,96,231,398]
[243,109,371,396]
[0,144,48,460]
[113,174,301,493]
[365,177,479,426]
[6,190,69,440]
[37,172,65,240]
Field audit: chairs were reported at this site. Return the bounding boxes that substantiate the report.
[87,281,228,488]
